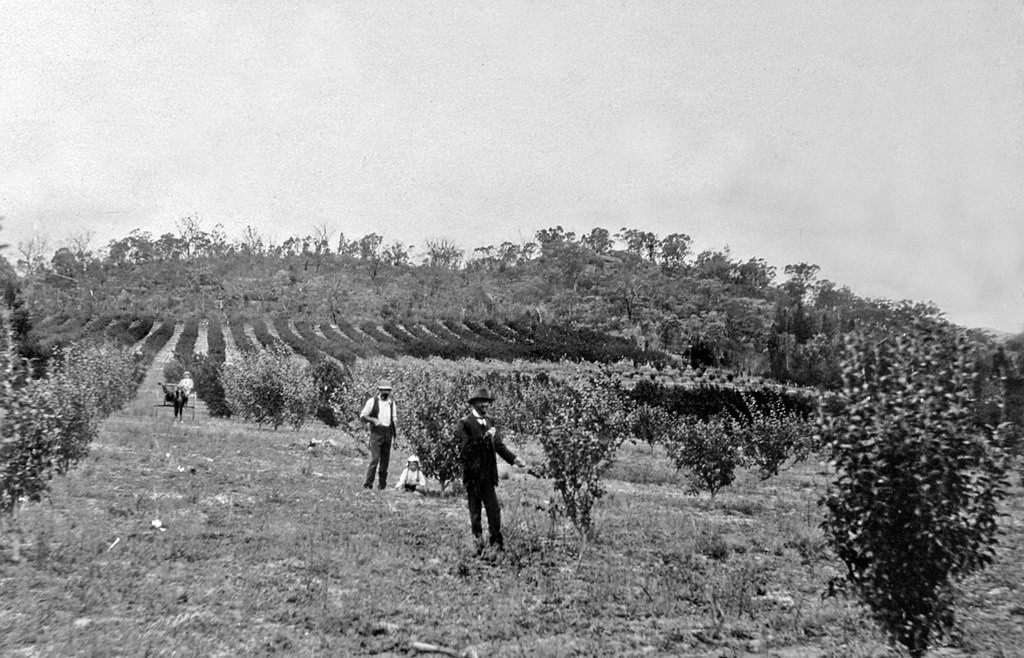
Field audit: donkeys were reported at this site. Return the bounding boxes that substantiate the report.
[162,385,188,424]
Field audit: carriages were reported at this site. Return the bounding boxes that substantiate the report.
[154,381,198,422]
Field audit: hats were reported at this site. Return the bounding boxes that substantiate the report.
[377,379,392,390]
[406,455,423,468]
[467,388,496,405]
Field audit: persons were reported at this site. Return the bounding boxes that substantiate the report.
[451,388,528,555]
[359,381,399,491]
[395,455,428,497]
[174,371,194,397]
